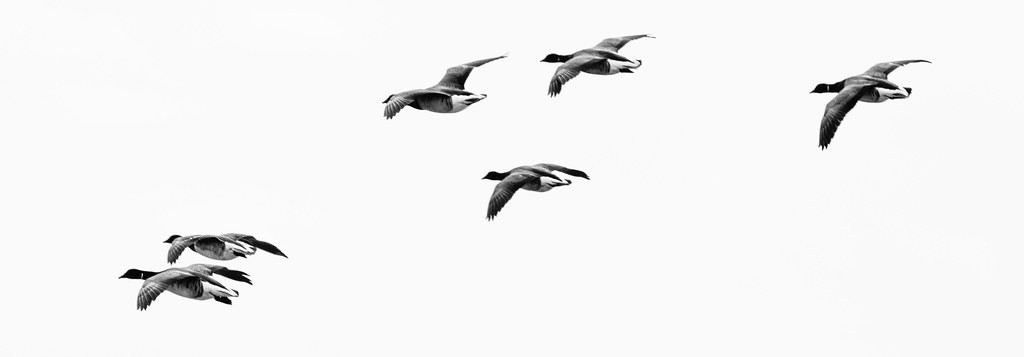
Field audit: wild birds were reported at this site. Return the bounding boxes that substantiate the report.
[481,163,592,222]
[540,35,656,98]
[810,60,932,151]
[118,233,290,311]
[381,55,508,119]
